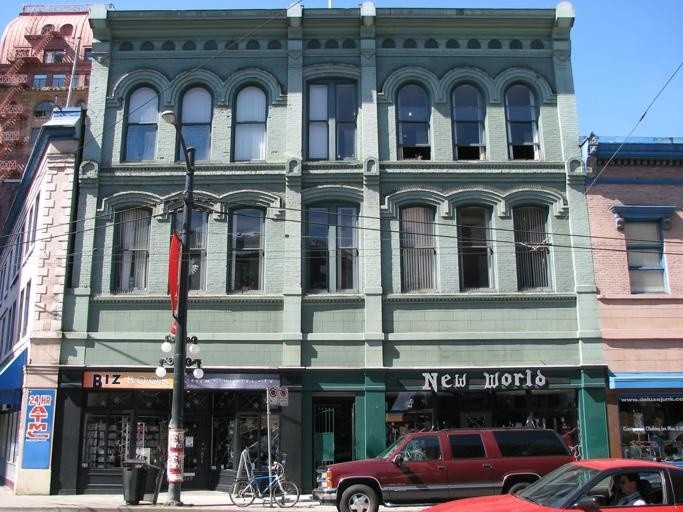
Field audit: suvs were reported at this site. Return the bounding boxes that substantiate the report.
[310,427,578,512]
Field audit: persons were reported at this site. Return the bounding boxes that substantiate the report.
[612,472,648,505]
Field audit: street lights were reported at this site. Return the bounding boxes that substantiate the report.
[155,106,205,505]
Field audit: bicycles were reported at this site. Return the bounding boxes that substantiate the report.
[229,460,299,508]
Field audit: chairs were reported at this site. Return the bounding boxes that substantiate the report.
[639,480,653,503]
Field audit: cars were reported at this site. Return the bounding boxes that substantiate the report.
[425,456,682,512]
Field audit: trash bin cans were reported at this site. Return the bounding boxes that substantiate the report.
[122,460,147,505]
